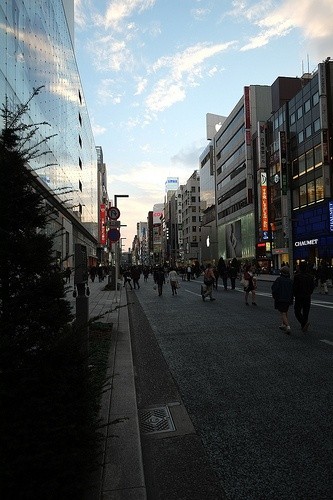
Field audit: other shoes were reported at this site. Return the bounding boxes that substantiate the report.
[202,295,205,301]
[301,323,311,333]
[279,323,291,332]
[210,298,216,301]
[244,302,258,307]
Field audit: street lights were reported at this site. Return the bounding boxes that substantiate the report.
[121,237,126,248]
[118,224,127,249]
[121,245,125,253]
[114,195,129,291]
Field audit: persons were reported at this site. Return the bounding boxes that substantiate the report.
[221,268,228,290]
[97,268,103,282]
[167,267,178,295]
[120,264,149,277]
[154,269,159,284]
[123,270,133,290]
[238,263,262,286]
[315,260,328,295]
[280,260,300,276]
[89,268,96,282]
[88,265,115,278]
[65,267,71,284]
[130,267,140,290]
[212,266,218,291]
[155,269,165,296]
[228,264,236,289]
[150,263,206,282]
[271,269,294,333]
[202,263,216,302]
[229,223,237,259]
[242,265,257,306]
[143,267,149,282]
[291,262,315,332]
